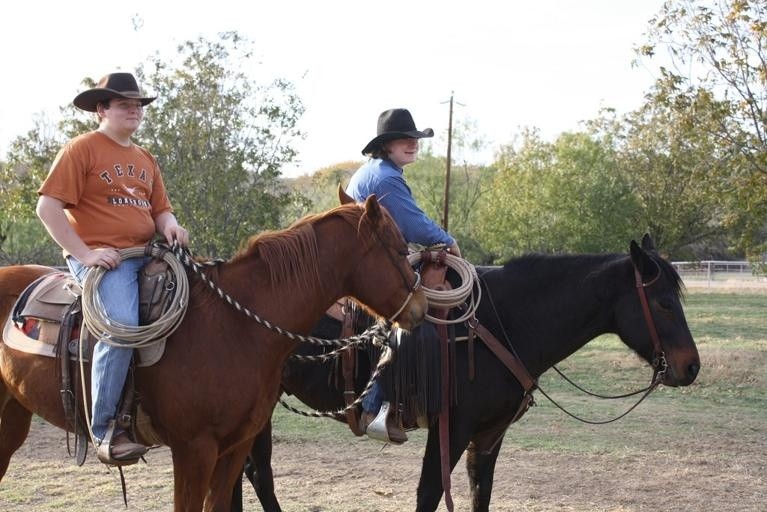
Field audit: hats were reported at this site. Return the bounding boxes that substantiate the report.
[361,108,434,155]
[73,72,157,112]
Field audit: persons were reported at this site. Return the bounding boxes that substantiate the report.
[342,106,463,445]
[33,72,191,462]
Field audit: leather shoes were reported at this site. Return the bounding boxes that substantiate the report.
[359,408,408,443]
[98,433,147,462]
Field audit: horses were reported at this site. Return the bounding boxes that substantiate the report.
[0,179,433,512]
[228,233,705,512]
